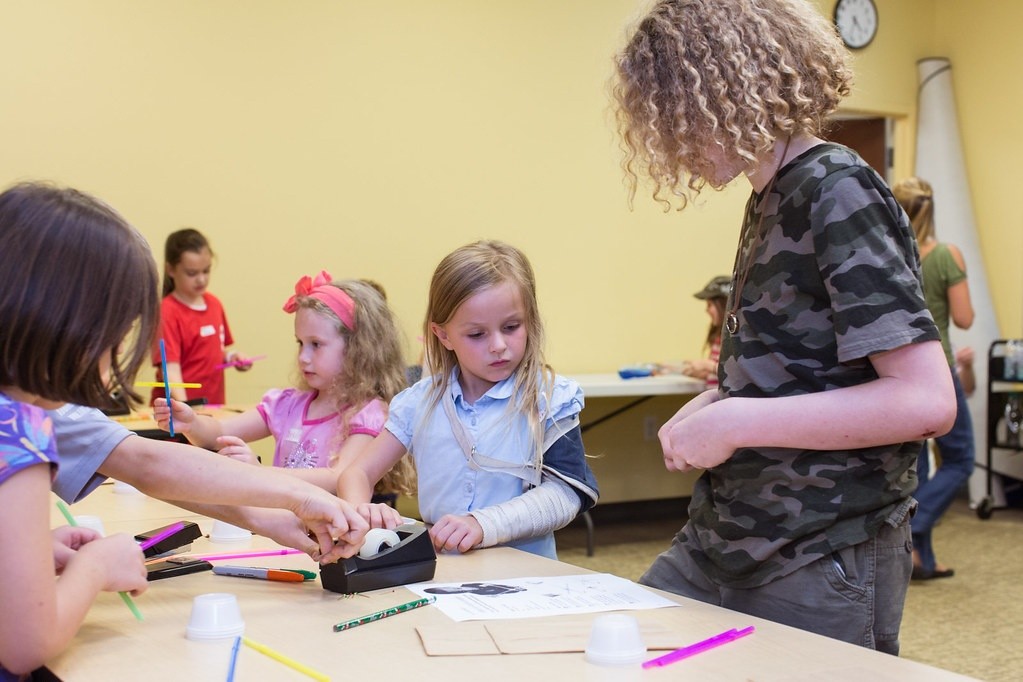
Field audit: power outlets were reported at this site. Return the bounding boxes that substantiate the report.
[642,416,657,442]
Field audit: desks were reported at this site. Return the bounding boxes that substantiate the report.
[107,403,252,440]
[41,478,983,682]
[567,373,707,557]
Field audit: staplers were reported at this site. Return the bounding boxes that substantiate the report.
[133,520,214,583]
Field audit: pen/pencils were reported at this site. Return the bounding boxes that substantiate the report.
[211,564,317,583]
[332,595,437,633]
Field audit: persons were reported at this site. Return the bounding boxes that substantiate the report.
[892,178,976,581]
[605,1,956,660]
[154,270,418,501]
[146,229,252,410]
[683,274,731,392]
[1,179,371,682]
[336,237,603,562]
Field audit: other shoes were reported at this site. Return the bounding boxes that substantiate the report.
[911,548,953,581]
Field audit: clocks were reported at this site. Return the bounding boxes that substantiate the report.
[833,0,880,50]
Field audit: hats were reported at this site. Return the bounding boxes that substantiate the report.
[694,274,734,301]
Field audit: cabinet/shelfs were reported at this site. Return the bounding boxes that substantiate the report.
[974,337,1023,522]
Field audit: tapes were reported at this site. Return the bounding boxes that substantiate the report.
[358,527,401,558]
